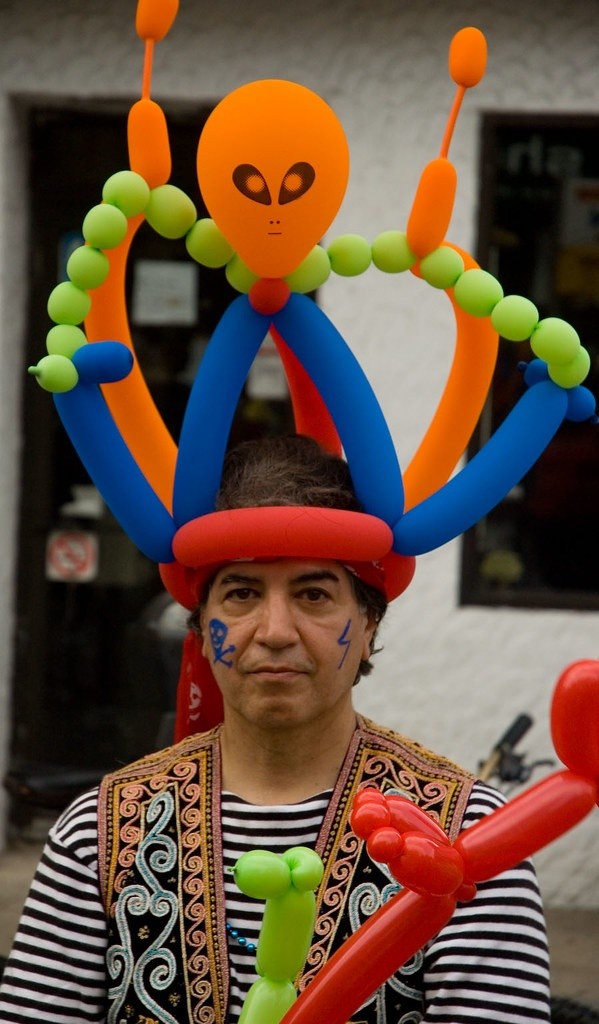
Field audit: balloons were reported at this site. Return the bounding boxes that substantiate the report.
[230,661,599,1024]
[28,0,594,611]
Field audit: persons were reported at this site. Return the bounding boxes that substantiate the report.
[0,435,550,1024]
